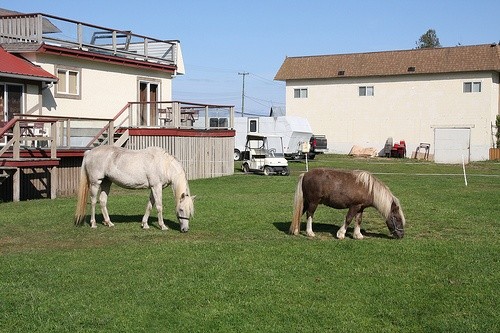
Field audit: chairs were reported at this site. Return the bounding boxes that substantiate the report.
[158,107,199,129]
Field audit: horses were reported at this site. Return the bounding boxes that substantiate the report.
[73,144,197,233]
[288,167,406,240]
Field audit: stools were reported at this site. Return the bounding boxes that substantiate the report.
[414,143,430,161]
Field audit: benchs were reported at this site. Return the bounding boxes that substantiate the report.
[251,148,268,159]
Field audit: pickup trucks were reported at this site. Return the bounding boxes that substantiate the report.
[291,135,328,160]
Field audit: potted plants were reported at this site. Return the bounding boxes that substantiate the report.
[490,114,500,160]
